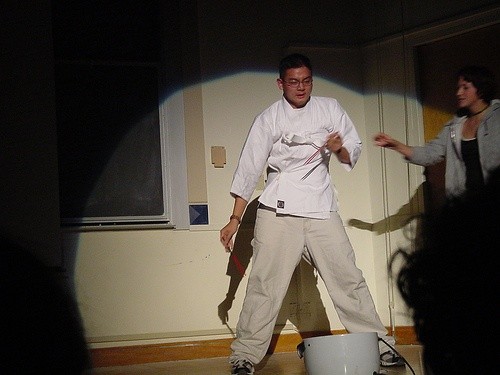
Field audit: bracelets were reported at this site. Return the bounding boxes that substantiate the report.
[230,215,241,224]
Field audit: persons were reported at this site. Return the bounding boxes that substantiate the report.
[371,72,500,201]
[219,55,405,375]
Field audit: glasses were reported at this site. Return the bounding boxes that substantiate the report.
[281,78,312,87]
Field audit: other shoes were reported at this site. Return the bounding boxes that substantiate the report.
[229,360,256,375]
[379,351,405,367]
